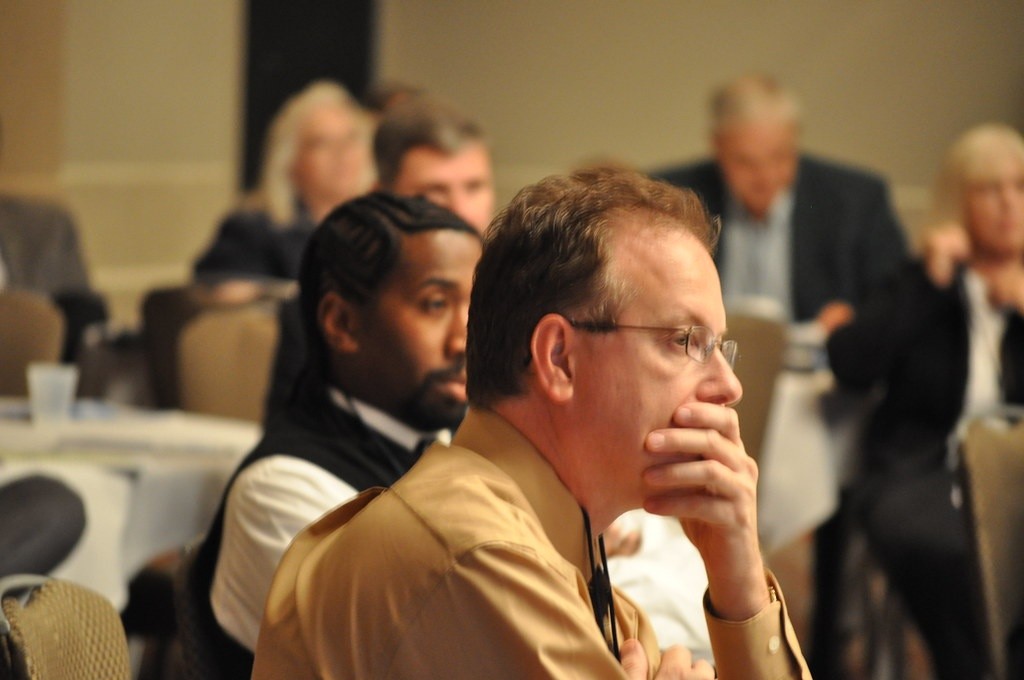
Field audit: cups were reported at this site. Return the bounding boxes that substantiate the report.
[26,362,81,423]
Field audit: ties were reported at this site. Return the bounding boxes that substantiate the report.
[587,564,612,642]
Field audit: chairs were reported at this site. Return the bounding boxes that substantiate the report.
[0,290,1023,680]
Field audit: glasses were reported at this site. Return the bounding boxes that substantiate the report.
[522,322,739,371]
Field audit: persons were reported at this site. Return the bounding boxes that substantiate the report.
[822,127,1023,680]
[250,170,816,680]
[190,78,379,310]
[647,73,910,680]
[370,102,492,240]
[187,190,485,680]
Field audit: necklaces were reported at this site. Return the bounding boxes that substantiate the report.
[579,508,621,665]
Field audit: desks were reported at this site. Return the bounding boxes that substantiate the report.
[1,392,260,605]
